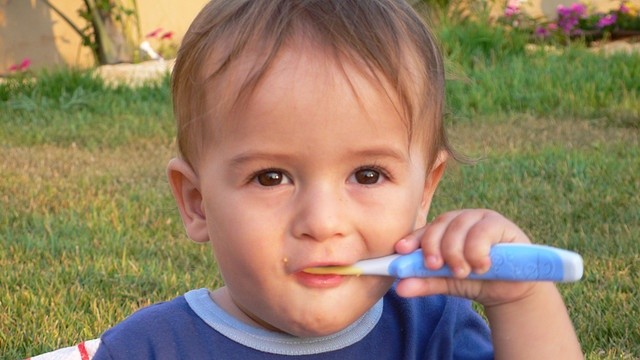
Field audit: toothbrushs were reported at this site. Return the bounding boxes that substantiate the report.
[302,243,584,285]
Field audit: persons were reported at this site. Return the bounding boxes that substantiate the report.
[91,0,588,360]
